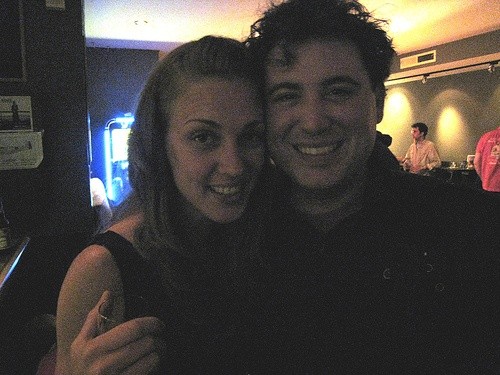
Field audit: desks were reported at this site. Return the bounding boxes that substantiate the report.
[430,167,483,184]
[0,236,30,292]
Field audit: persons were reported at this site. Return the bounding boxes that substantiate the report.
[55,36,390,375]
[36,0,500,375]
[403,122,441,176]
[473,127,500,212]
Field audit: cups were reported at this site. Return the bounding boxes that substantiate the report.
[95,295,150,334]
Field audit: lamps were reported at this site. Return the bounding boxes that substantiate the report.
[488,63,495,73]
[422,74,430,84]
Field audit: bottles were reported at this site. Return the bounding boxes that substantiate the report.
[0,202,11,255]
[466,155,475,170]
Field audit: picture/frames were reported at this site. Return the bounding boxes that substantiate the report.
[0,0,27,83]
